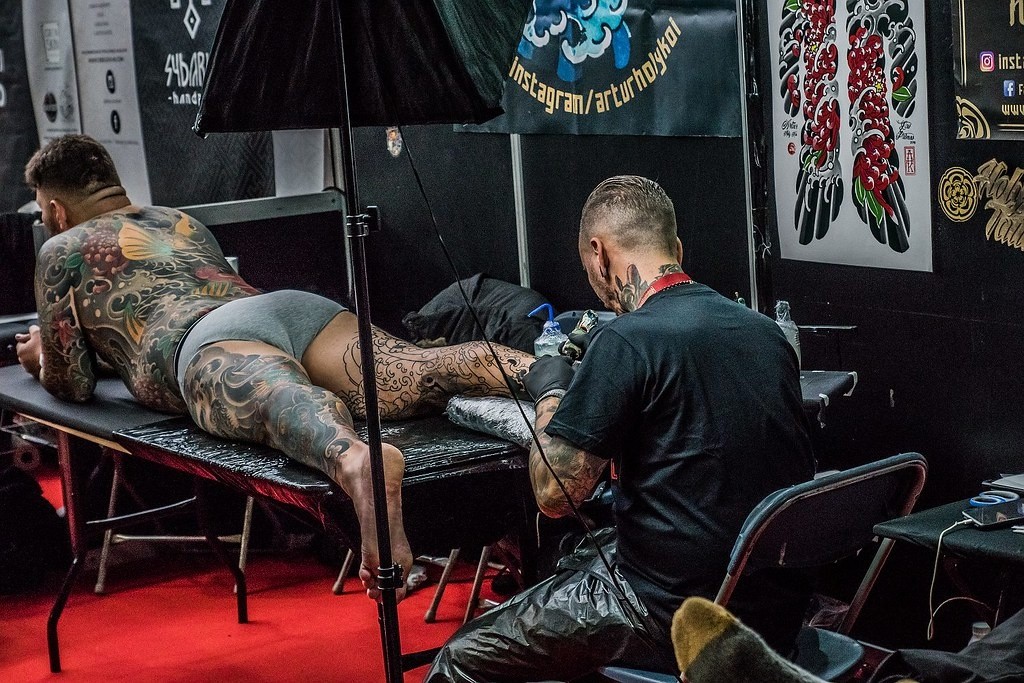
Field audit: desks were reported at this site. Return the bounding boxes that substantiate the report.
[870,480,1023,658]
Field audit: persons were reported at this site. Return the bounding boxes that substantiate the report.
[15,132,541,603]
[421,174,816,683]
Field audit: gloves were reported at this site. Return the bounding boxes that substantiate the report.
[523,355,576,407]
[557,323,605,362]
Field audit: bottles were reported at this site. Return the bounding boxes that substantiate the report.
[774,300,803,376]
[533,321,567,356]
[969,622,991,647]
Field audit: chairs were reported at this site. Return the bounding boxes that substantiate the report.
[596,451,928,683]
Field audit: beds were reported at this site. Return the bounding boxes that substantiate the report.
[1,360,608,624]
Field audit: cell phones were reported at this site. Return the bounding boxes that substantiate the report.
[962,498,1024,530]
